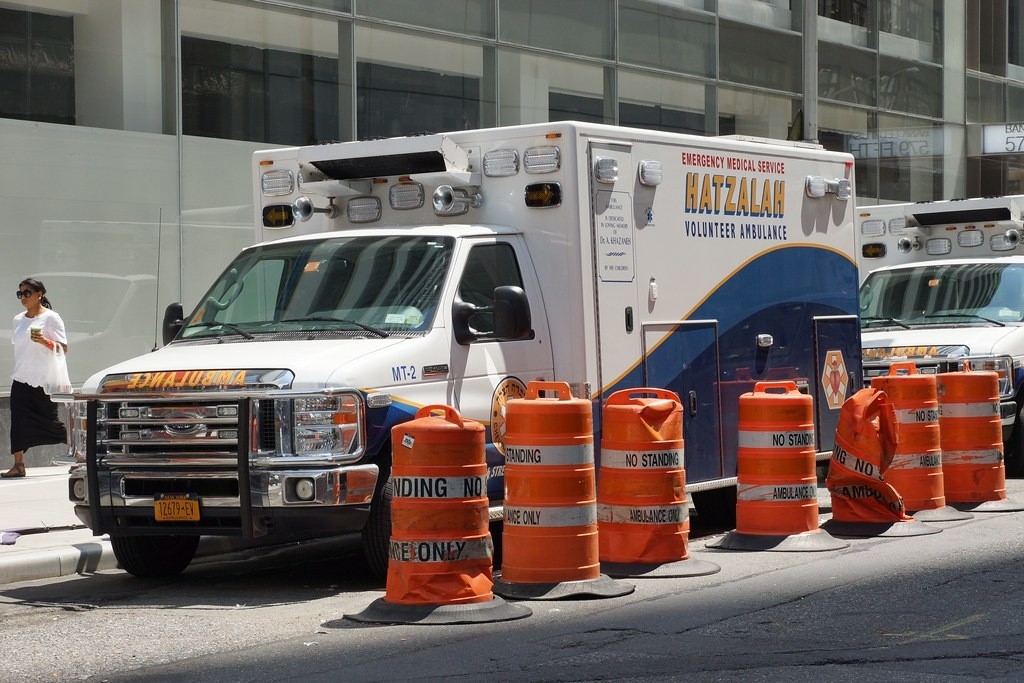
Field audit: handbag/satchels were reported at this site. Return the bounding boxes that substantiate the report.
[43,341,74,395]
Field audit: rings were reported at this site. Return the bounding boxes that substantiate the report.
[36,339,38,341]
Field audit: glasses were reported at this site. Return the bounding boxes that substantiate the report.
[16,288,36,299]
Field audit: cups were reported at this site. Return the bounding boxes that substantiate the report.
[30,324,42,341]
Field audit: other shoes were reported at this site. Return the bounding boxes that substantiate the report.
[1,463,25,478]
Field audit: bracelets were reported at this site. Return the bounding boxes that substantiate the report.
[48,340,54,349]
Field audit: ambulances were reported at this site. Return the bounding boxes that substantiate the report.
[49,120,864,590]
[857,192,1024,478]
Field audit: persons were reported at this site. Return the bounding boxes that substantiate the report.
[0,278,68,477]
